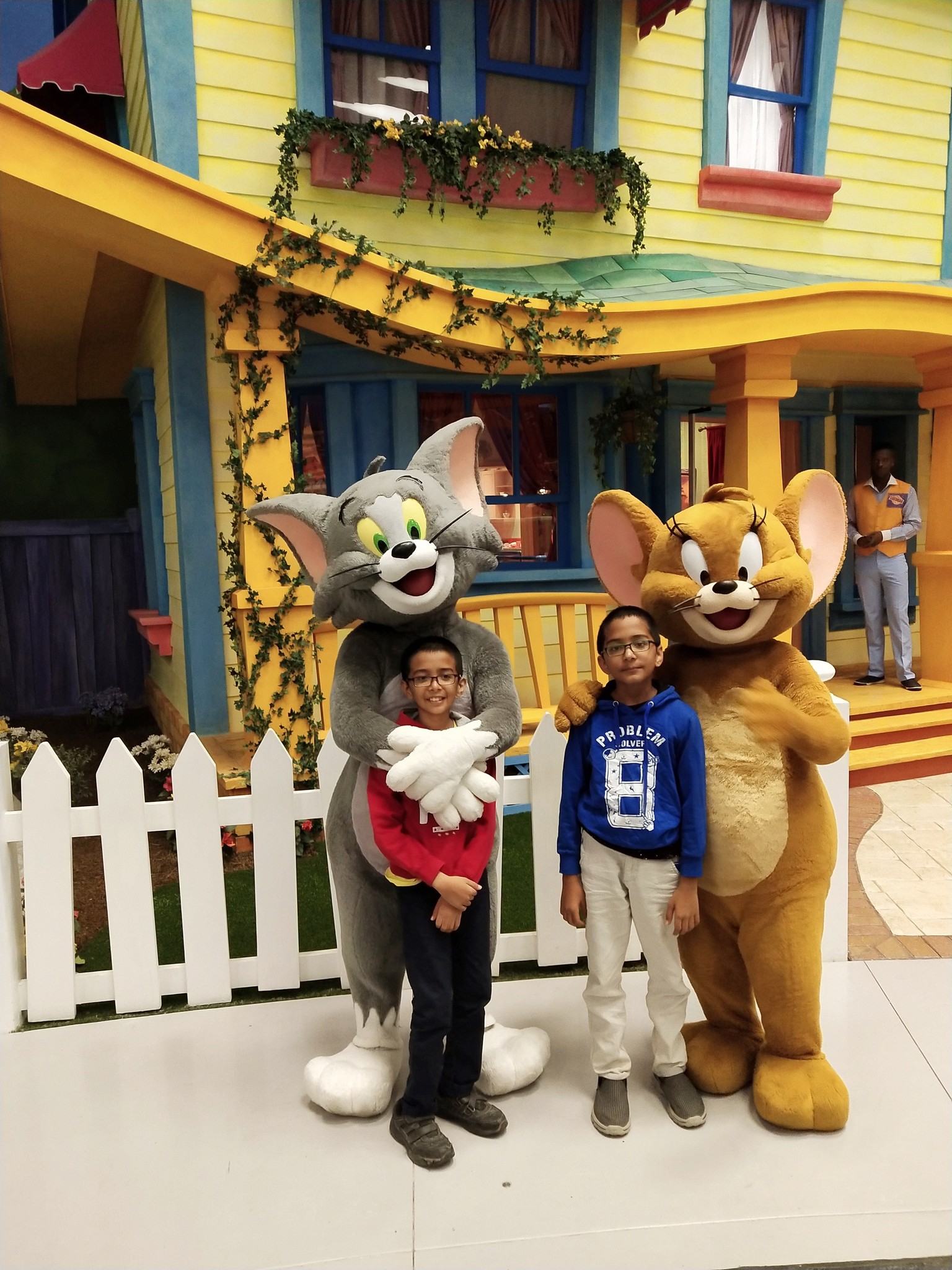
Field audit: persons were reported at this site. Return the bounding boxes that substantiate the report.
[556,606,707,1138]
[847,445,923,692]
[367,638,509,1169]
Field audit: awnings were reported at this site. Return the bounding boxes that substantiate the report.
[16,0,125,141]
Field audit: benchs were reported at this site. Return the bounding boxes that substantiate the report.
[304,587,667,776]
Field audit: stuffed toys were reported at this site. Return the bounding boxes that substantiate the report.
[554,468,849,1133]
[244,417,551,1116]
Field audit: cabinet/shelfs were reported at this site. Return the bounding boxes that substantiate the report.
[479,465,550,563]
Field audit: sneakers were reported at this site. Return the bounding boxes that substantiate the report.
[590,1077,631,1136]
[854,675,886,685]
[900,678,922,690]
[432,1083,508,1138]
[389,1098,455,1168]
[652,1072,707,1127]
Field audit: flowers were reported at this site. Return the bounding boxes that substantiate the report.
[279,109,648,259]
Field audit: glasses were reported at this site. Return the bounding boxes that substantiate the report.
[406,673,461,687]
[600,639,658,657]
